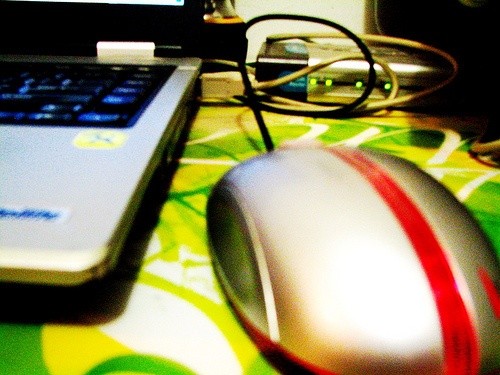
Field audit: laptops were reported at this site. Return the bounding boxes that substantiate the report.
[1,0,210,314]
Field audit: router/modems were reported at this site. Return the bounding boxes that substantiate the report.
[255,40,447,102]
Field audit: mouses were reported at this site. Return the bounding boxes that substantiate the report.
[205,145,500,375]
